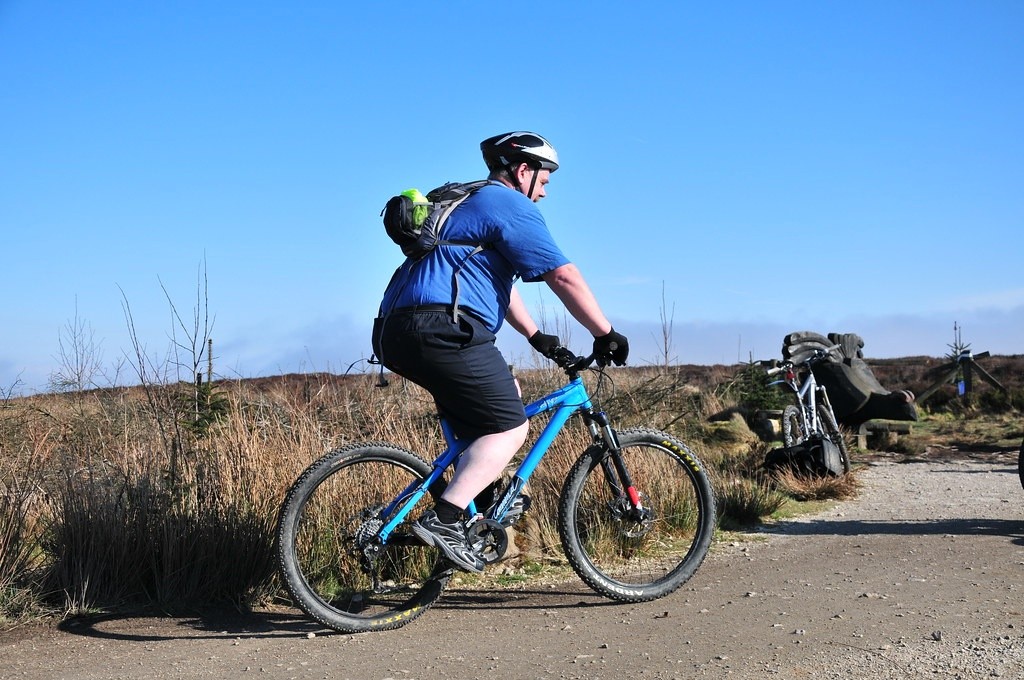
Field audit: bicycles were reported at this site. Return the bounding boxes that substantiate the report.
[273,339,717,633]
[766,343,849,480]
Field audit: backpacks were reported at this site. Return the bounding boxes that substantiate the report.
[380,178,490,258]
[765,433,841,480]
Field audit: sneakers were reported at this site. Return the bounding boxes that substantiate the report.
[412,511,484,573]
[485,494,530,518]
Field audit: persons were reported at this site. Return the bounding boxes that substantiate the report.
[371,131,629,573]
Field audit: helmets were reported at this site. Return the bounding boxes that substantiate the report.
[481,131,560,173]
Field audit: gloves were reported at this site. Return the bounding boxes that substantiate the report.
[594,327,629,368]
[528,330,561,360]
